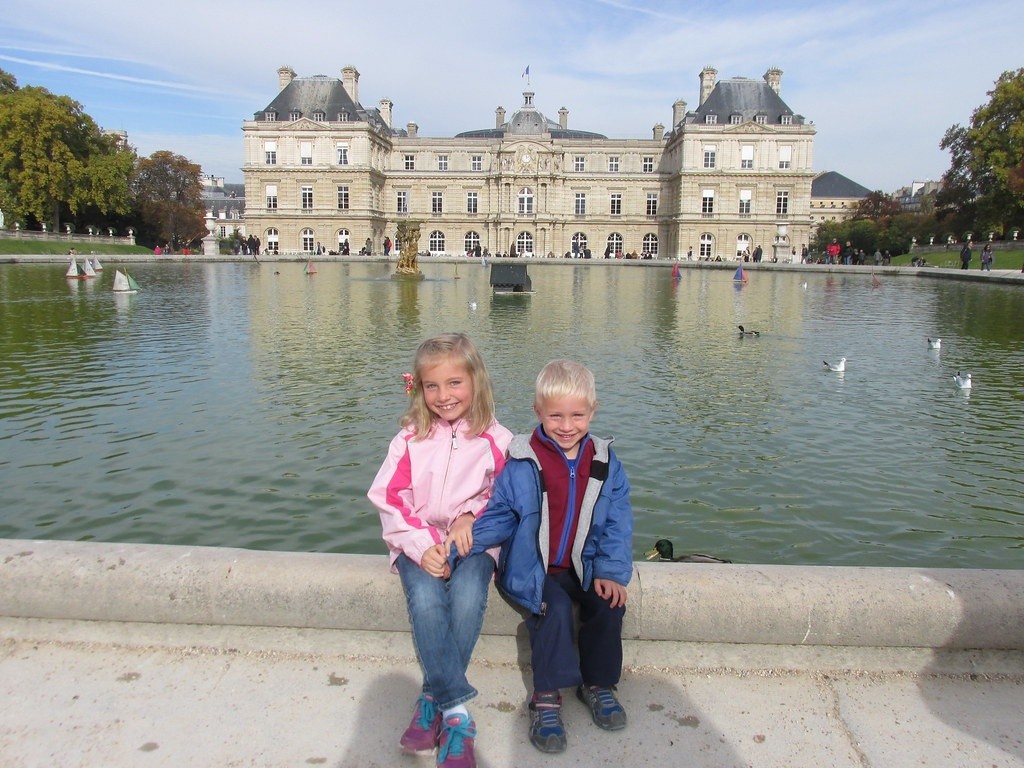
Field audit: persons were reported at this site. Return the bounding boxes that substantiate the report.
[234,240,240,255]
[467,243,488,257]
[980,244,992,271]
[366,333,514,768]
[960,241,973,269]
[315,242,323,255]
[262,248,278,255]
[742,245,762,262]
[254,235,260,255]
[421,250,430,256]
[801,244,808,264]
[68,248,76,255]
[548,238,583,258]
[248,235,255,255]
[342,245,348,255]
[154,245,161,255]
[705,255,721,262]
[604,245,652,259]
[165,244,170,254]
[687,246,692,260]
[911,256,926,267]
[496,251,509,257]
[241,236,248,255]
[365,238,372,256]
[441,359,633,754]
[383,237,391,256]
[824,238,890,265]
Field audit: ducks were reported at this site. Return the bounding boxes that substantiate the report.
[736,325,759,335]
[643,539,733,564]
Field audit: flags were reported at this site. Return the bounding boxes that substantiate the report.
[522,66,529,77]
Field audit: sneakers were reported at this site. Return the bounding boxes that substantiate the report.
[531,691,567,754]
[399,698,440,754]
[438,711,477,767]
[577,682,628,732]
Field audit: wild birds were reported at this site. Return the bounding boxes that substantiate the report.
[823,358,847,372]
[952,372,972,388]
[928,338,941,348]
[468,301,477,307]
